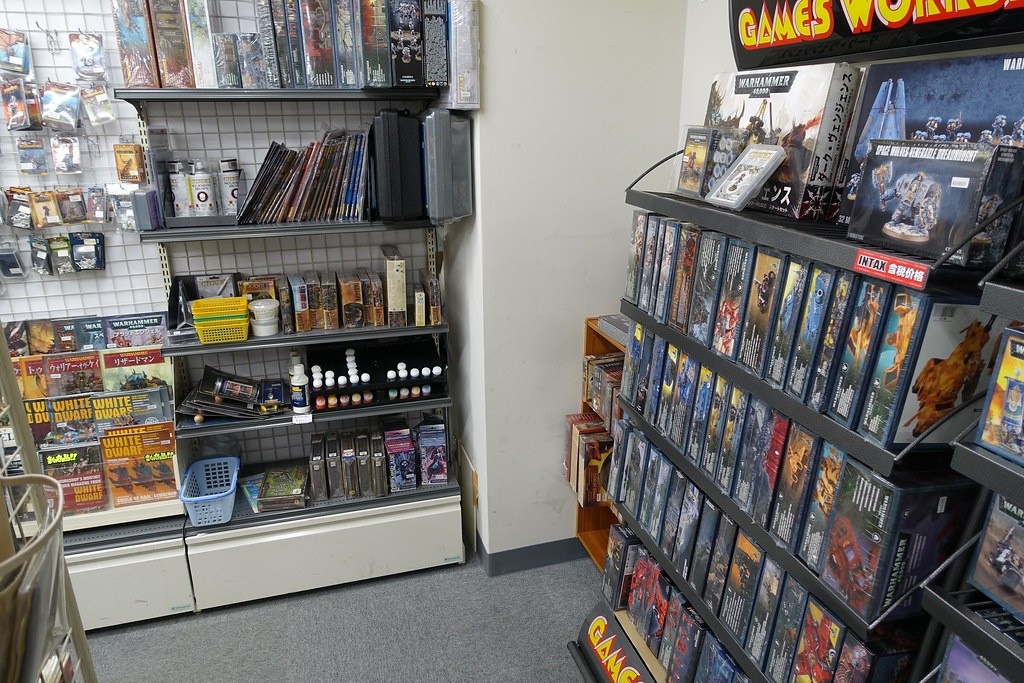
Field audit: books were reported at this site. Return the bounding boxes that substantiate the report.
[238,129,368,224]
[238,244,443,334]
[0,312,178,514]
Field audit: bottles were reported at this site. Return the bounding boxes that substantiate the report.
[167,157,242,217]
[288,348,311,415]
[311,347,443,409]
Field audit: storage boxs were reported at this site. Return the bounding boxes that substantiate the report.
[131,189,159,231]
[368,108,472,226]
[565,353,623,510]
[109,0,482,110]
[257,415,448,513]
[598,209,1024,683]
[673,53,1024,270]
[191,244,442,345]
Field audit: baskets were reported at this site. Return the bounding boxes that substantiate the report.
[179,456,240,527]
[191,297,249,344]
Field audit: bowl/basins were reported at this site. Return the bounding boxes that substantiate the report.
[247,298,280,320]
[249,317,279,337]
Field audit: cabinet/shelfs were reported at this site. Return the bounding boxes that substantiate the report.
[0,310,195,631]
[576,315,626,575]
[919,241,1024,683]
[567,0,1024,683]
[114,86,465,611]
